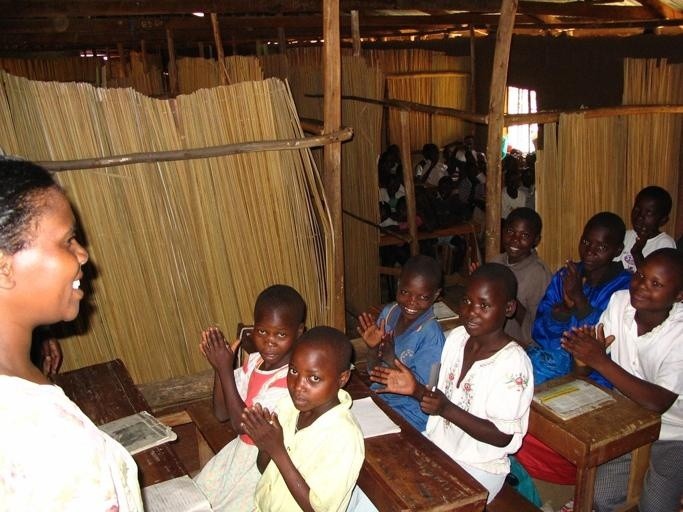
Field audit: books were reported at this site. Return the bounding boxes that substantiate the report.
[97,410,178,457]
[348,395,403,440]
[533,378,617,422]
[140,474,214,511]
[432,300,460,324]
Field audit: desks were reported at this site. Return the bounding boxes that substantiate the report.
[376,172,480,274]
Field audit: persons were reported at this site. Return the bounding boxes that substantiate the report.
[471,206,554,353]
[241,324,366,511]
[371,263,535,505]
[356,254,446,433]
[1,157,146,512]
[378,133,537,302]
[560,246,683,511]
[612,185,678,275]
[523,211,635,511]
[199,284,308,512]
